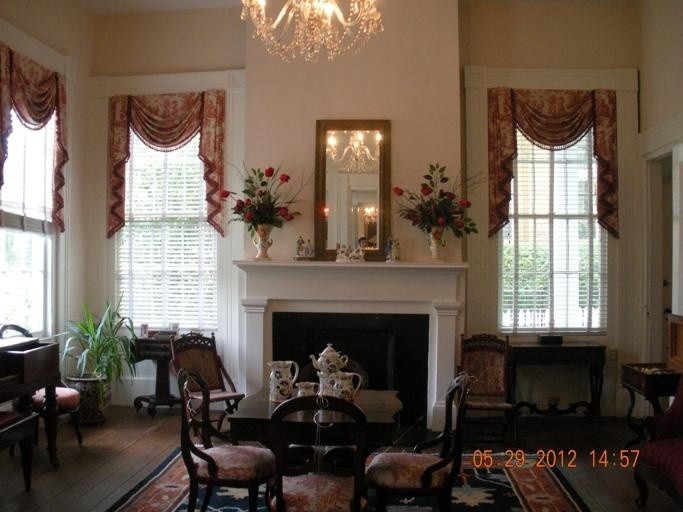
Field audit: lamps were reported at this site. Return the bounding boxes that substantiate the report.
[326,130,382,171]
[359,203,377,224]
[240,1,384,66]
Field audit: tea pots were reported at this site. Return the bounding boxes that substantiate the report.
[309,343,349,376]
[296,381,320,398]
[317,371,366,416]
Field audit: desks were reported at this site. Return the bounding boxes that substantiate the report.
[1,337,62,473]
[130,335,179,417]
[227,381,403,512]
[509,337,607,432]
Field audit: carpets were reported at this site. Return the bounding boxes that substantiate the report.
[106,446,592,512]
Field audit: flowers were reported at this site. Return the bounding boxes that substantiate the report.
[215,158,314,243]
[391,157,476,246]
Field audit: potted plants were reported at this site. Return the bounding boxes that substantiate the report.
[58,290,138,427]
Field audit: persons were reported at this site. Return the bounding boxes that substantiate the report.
[358,237,369,248]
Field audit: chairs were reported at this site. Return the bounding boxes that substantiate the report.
[455,331,517,447]
[177,366,277,512]
[264,395,367,512]
[365,373,470,511]
[1,410,39,490]
[0,323,83,469]
[171,330,246,440]
[625,380,682,510]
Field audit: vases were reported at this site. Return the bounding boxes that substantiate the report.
[255,222,273,259]
[428,225,443,261]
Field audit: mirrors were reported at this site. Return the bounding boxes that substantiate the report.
[313,119,393,264]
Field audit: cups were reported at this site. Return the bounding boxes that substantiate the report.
[267,361,300,402]
